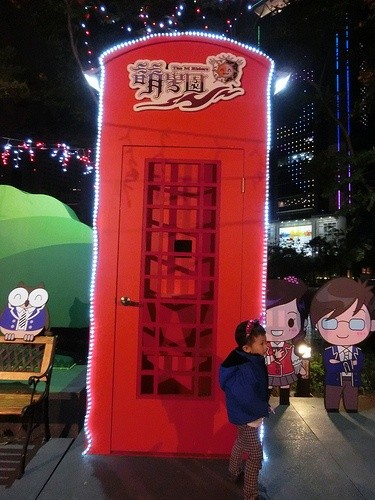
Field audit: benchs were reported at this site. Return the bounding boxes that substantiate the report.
[0,336,58,479]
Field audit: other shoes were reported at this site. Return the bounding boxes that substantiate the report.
[255,494,266,500]
[237,472,245,480]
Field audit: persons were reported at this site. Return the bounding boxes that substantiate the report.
[219,313,276,500]
